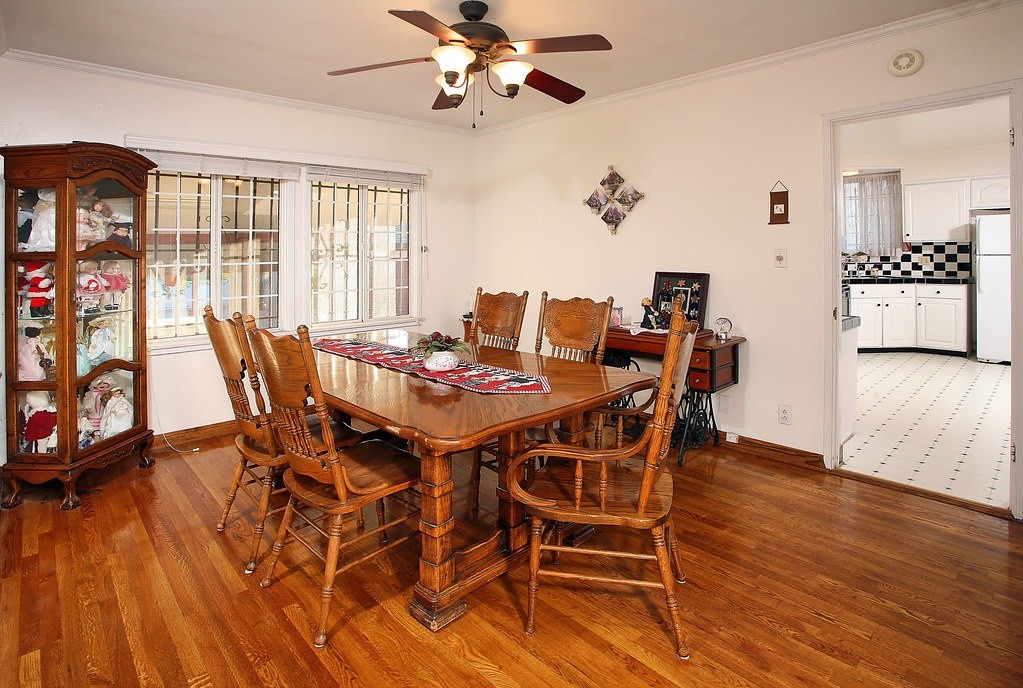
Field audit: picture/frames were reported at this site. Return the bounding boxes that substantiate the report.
[652,272,710,330]
[610,306,622,327]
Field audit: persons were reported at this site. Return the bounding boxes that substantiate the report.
[677,290,686,310]
[640,297,659,329]
[17,183,135,457]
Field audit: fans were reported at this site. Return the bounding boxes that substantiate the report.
[327,1,612,110]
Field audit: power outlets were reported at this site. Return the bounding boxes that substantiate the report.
[779,404,791,426]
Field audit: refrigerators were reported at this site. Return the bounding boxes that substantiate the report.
[972,214,1011,363]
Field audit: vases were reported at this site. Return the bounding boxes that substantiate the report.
[423,351,460,373]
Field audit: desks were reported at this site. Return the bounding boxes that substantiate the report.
[584,325,747,467]
[247,329,657,632]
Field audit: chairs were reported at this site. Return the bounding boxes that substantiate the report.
[242,314,421,648]
[472,291,614,511]
[508,298,690,659]
[406,288,529,498]
[203,306,367,573]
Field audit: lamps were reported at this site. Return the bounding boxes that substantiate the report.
[431,45,535,108]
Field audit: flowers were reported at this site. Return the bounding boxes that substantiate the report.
[409,331,471,361]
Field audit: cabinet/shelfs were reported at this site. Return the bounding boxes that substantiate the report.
[901,174,1012,243]
[839,281,971,465]
[0,140,158,510]
